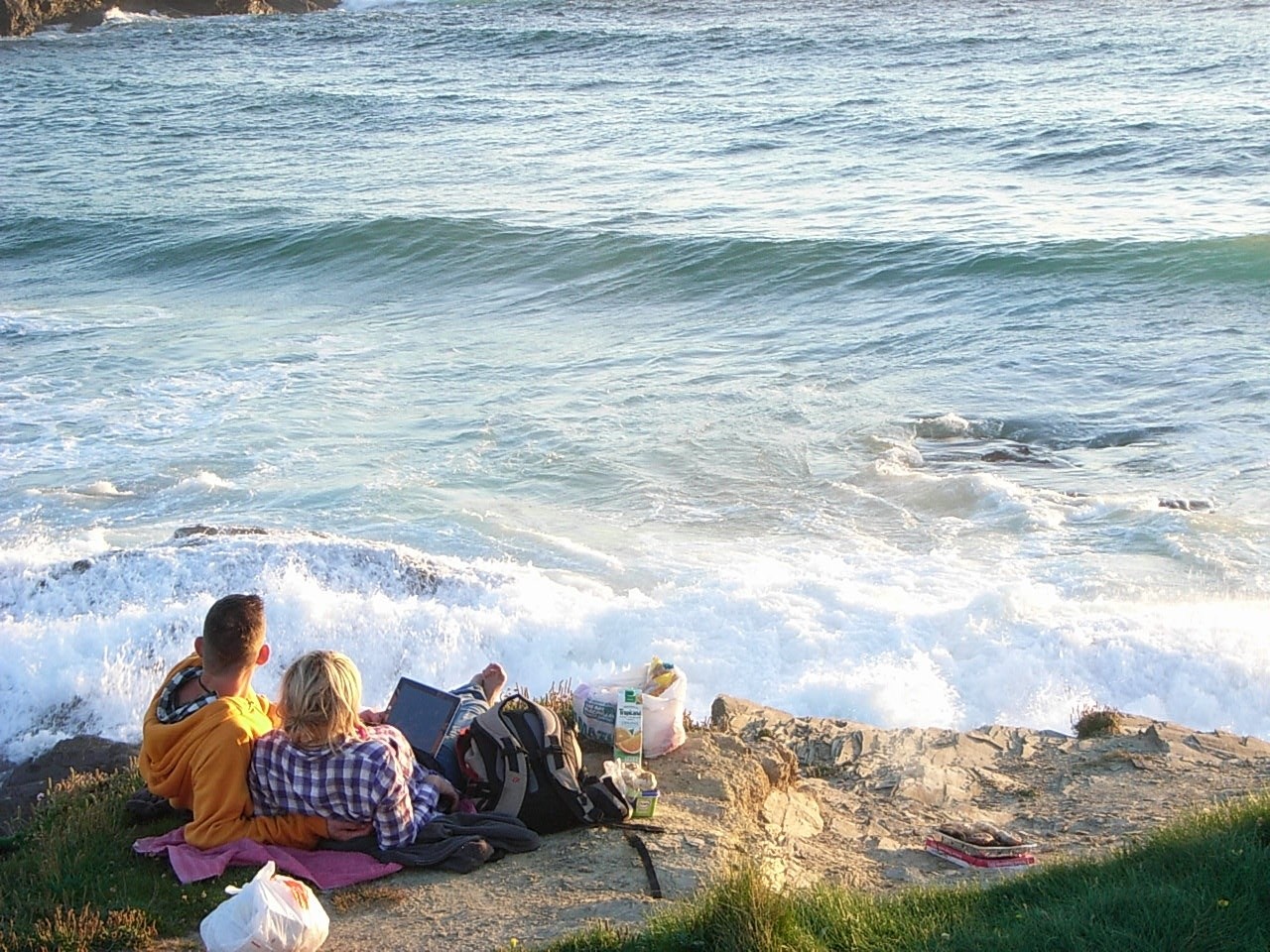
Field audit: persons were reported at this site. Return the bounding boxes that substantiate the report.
[245,650,506,851]
[138,593,374,849]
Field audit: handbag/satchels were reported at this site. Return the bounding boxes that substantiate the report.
[570,655,686,759]
[199,860,330,952]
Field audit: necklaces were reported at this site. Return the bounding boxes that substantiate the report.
[198,677,211,693]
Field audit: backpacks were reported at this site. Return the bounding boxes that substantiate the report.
[467,694,631,836]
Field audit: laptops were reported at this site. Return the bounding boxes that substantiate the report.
[380,676,461,760]
